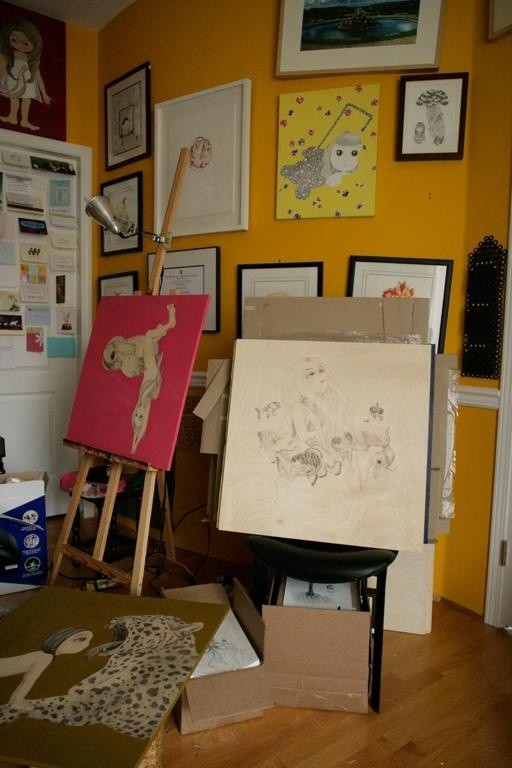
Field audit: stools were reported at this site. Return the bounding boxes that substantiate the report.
[55,465,151,578]
[245,534,400,713]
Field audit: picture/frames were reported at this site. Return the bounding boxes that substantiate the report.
[485,0,512,45]
[152,77,253,239]
[392,70,470,161]
[235,260,323,339]
[345,253,455,353]
[96,269,139,306]
[146,244,221,333]
[103,59,153,171]
[99,171,144,256]
[270,0,444,79]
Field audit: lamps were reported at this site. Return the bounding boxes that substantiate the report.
[81,193,174,251]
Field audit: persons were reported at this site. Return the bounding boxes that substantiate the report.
[287,354,335,442]
[129,302,178,456]
[1,625,94,712]
[1,18,53,134]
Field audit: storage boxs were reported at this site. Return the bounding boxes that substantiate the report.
[261,570,373,714]
[159,574,270,733]
[0,466,51,589]
